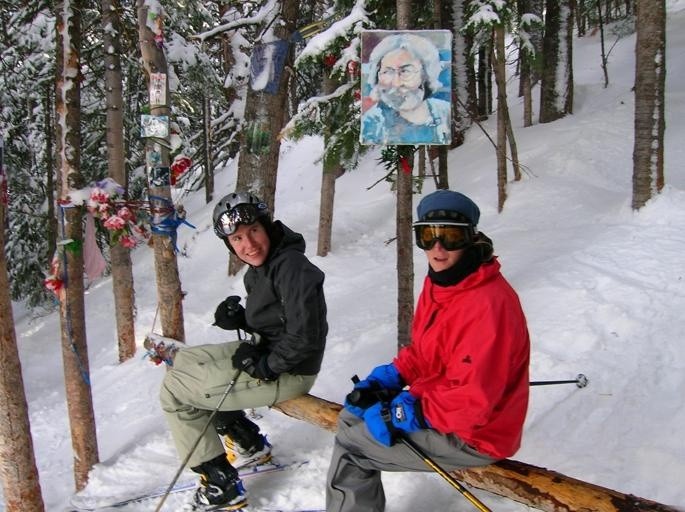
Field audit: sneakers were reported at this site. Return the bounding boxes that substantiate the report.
[188,474,250,511]
[225,435,273,469]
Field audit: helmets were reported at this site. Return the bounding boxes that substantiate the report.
[213,193,275,256]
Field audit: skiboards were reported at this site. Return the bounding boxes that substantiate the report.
[71,459,306,511]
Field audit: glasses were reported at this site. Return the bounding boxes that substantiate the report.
[412,222,472,250]
[214,202,267,236]
[376,67,422,82]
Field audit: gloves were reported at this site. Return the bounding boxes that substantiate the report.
[214,301,246,330]
[344,363,403,417]
[231,342,266,381]
[365,391,432,448]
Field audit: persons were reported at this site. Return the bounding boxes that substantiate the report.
[158,190,329,511]
[324,190,532,511]
[361,34,450,144]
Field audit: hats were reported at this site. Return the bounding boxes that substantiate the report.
[417,190,480,226]
